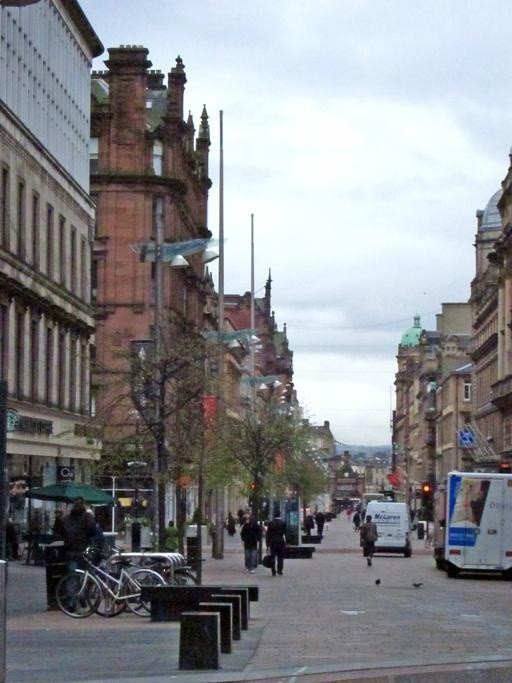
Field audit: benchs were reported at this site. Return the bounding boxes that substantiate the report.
[283,547,316,559]
[139,585,260,621]
[301,534,323,544]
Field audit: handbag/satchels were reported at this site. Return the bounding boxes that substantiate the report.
[263,555,274,568]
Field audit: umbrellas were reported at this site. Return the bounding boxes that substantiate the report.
[24,479,122,515]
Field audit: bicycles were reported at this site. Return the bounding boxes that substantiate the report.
[55,542,202,617]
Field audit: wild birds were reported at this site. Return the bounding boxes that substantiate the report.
[375,577,381,585]
[412,583,422,588]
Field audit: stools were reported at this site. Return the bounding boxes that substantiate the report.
[179,612,222,670]
[199,602,233,654]
[221,588,249,630]
[211,594,241,640]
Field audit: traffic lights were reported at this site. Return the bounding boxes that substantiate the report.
[422,480,431,493]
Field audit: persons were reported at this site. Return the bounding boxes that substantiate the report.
[305,512,315,535]
[164,519,179,552]
[52,497,98,612]
[359,514,378,565]
[86,506,106,564]
[315,510,324,539]
[460,479,491,528]
[227,506,285,576]
[352,509,361,532]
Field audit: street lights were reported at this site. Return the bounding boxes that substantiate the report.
[202,317,264,564]
[246,373,330,520]
[136,229,223,562]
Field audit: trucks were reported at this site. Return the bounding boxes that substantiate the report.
[431,468,512,579]
[358,490,417,560]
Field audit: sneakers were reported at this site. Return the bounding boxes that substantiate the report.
[366,556,373,566]
[245,567,257,574]
